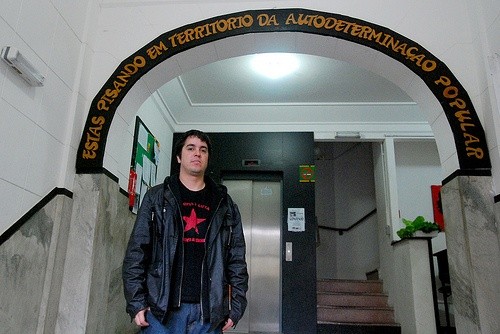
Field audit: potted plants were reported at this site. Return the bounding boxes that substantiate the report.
[396,216,441,238]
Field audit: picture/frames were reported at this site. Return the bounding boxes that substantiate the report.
[431,184,446,232]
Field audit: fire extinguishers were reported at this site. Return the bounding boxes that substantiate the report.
[128,165,137,207]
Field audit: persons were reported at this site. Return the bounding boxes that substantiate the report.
[122,129,249,334]
